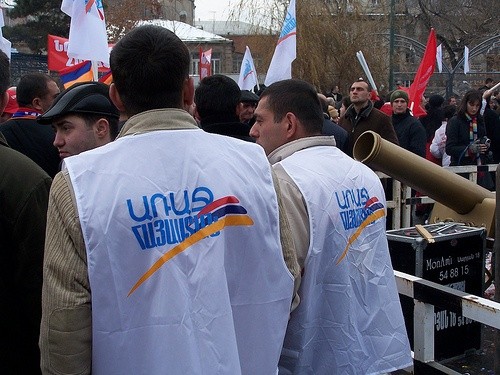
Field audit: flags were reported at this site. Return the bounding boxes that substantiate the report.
[264,0,297,87]
[436,43,442,73]
[464,46,469,74]
[238,45,256,91]
[60,59,95,90]
[61,0,110,66]
[409,28,436,118]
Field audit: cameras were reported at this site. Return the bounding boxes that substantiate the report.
[480,135,491,150]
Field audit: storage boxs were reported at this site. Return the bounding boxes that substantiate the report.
[386,222,488,363]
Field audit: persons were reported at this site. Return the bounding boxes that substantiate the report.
[0,50,127,375]
[249,79,413,375]
[39,26,302,375]
[192,74,500,230]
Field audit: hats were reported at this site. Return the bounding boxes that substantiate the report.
[425,94,450,112]
[390,90,409,104]
[35,81,120,125]
[3,86,19,114]
[317,93,328,112]
[240,89,259,102]
